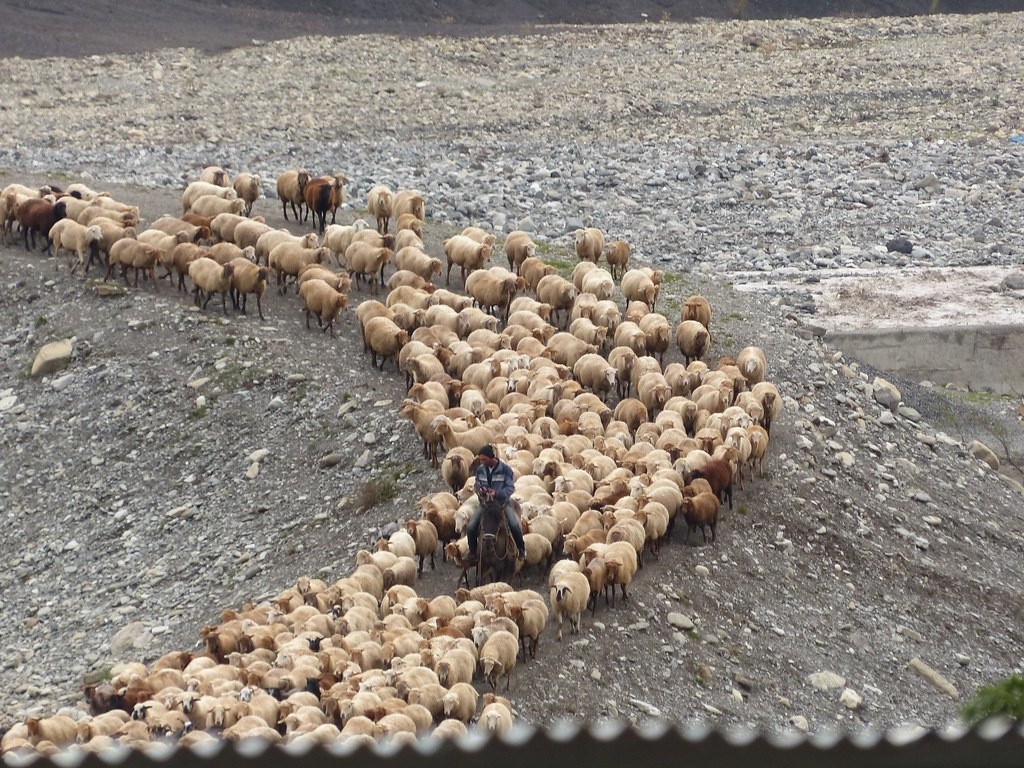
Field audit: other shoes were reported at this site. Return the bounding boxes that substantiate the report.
[519,550,527,562]
[462,552,478,561]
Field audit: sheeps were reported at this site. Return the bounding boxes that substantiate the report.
[0,160,784,758]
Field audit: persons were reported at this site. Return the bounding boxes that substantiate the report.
[466,445,525,561]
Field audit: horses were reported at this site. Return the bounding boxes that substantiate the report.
[475,496,524,592]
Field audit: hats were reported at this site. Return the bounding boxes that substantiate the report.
[478,444,495,458]
